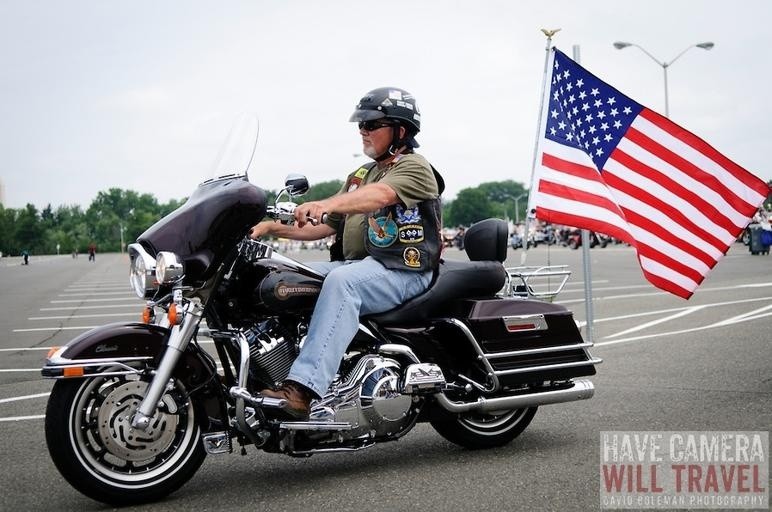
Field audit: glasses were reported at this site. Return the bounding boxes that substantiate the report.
[358,120,395,130]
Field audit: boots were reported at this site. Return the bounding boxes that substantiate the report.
[260,383,311,422]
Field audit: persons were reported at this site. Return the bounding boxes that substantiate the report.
[87,241,96,261]
[21,250,30,265]
[251,87,445,421]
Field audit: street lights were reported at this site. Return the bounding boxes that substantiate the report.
[613,41,714,117]
[503,192,528,222]
[120,207,136,253]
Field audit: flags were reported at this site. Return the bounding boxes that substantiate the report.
[532,48,772,300]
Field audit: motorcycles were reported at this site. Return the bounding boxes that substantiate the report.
[41,173,603,507]
[508,221,612,249]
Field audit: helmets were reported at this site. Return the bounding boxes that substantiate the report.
[348,87,420,132]
